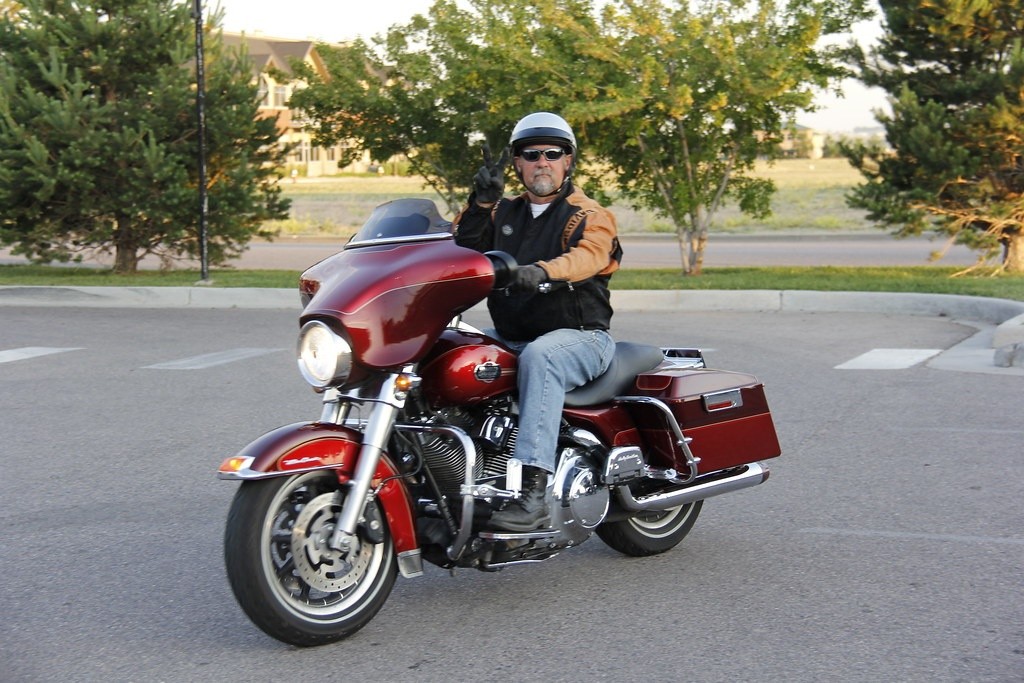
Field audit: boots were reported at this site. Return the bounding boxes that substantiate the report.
[485,466,552,532]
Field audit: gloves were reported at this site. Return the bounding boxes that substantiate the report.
[514,263,548,298]
[472,143,510,204]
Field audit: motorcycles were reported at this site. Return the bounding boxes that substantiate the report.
[216,196,781,648]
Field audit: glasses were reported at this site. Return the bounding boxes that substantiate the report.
[519,147,566,162]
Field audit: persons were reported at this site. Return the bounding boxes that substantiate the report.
[453,112,623,532]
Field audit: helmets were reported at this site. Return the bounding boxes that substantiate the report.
[509,112,578,156]
